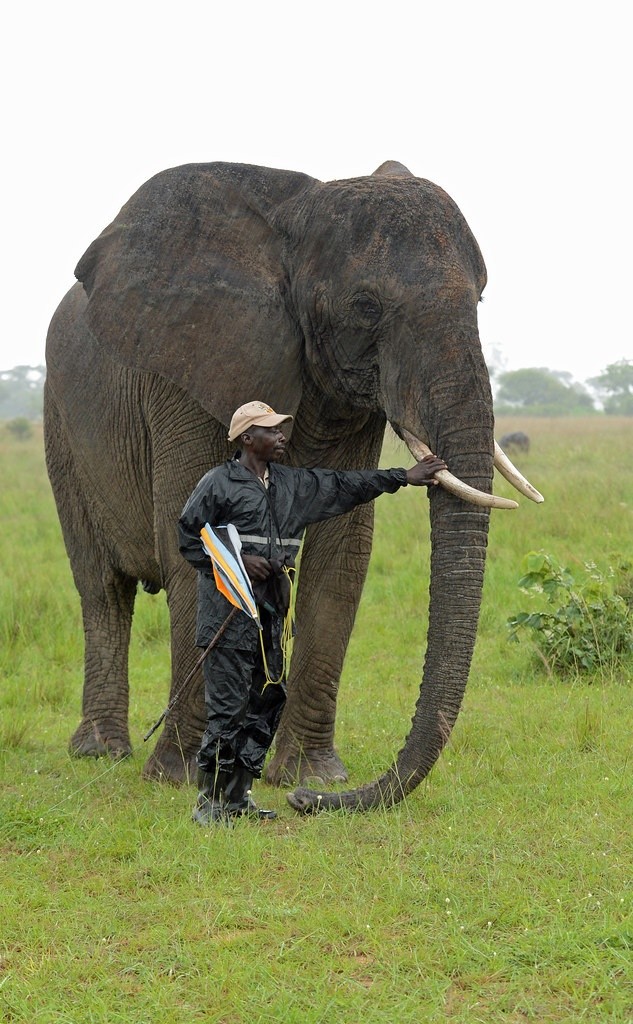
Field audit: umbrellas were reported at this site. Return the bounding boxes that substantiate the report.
[199,522,263,631]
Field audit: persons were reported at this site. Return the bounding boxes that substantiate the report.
[176,400,449,831]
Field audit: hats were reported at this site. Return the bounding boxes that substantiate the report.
[227,401,294,443]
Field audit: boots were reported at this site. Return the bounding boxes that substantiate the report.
[222,764,277,819]
[191,767,232,827]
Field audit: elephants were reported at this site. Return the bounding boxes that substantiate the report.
[41,161,543,817]
